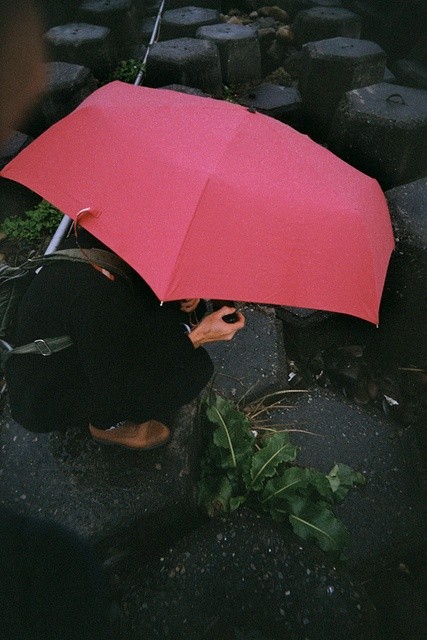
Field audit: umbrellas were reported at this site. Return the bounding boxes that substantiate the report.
[0,80,396,329]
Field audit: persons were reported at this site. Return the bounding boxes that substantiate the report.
[0,229,246,453]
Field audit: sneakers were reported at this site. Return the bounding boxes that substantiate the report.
[89,418,171,452]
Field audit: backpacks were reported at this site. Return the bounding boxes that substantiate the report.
[1,248,133,398]
